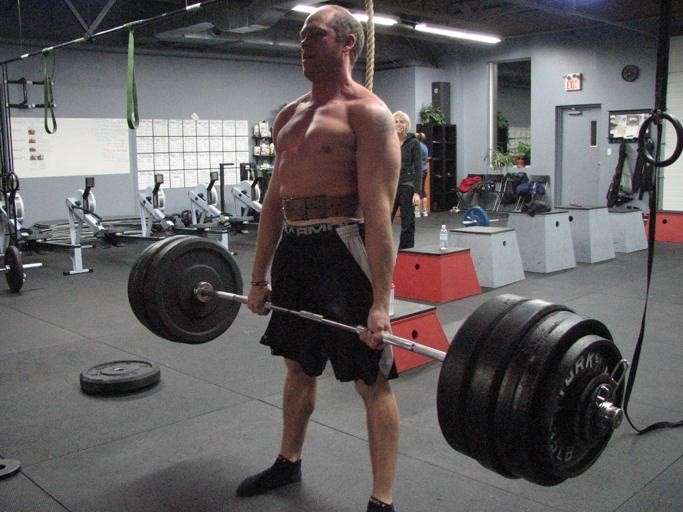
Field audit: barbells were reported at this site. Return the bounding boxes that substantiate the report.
[462,206,509,227]
[128,235,624,487]
[0,245,43,293]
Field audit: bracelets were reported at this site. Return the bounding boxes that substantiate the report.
[248,279,269,287]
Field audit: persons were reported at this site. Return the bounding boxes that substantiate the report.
[390,109,422,250]
[412,131,429,219]
[236,4,403,511]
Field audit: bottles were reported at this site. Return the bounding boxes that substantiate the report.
[439,224,448,250]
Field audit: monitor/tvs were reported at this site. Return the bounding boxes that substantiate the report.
[607,108,653,144]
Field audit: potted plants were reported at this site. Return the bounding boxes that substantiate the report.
[514,142,532,169]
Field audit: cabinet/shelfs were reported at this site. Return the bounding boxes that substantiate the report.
[251,127,274,179]
[415,122,456,212]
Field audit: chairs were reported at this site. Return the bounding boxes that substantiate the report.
[450,173,551,216]
